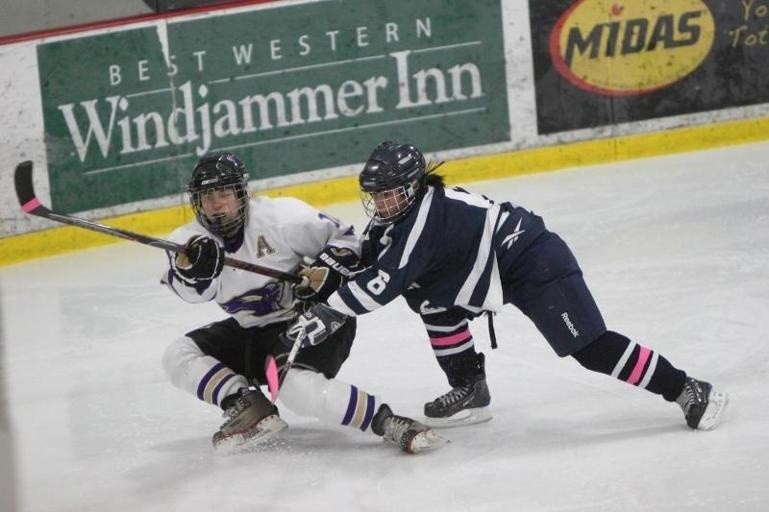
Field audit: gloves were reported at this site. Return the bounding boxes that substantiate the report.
[283,303,350,348]
[290,245,360,304]
[171,234,226,291]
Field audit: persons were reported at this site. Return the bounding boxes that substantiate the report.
[279,141,716,429]
[161,153,431,450]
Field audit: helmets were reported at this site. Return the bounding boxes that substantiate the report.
[188,150,251,207]
[357,140,429,201]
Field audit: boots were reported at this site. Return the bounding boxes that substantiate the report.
[369,403,434,454]
[674,373,713,428]
[419,352,492,417]
[211,388,281,445]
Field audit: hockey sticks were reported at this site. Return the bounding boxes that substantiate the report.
[15,161,312,288]
[265,320,306,405]
